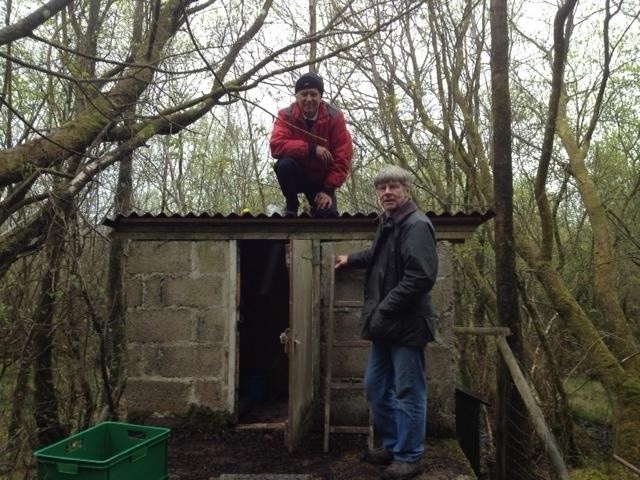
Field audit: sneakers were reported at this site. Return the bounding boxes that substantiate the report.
[285,201,299,217]
[358,446,393,464]
[378,456,424,480]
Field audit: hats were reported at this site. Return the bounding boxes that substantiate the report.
[295,72,324,94]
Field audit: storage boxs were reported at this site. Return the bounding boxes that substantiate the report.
[35,422,172,479]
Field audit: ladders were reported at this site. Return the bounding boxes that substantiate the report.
[324,255,374,453]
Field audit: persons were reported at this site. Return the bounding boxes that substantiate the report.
[334,165,439,480]
[268,73,354,217]
